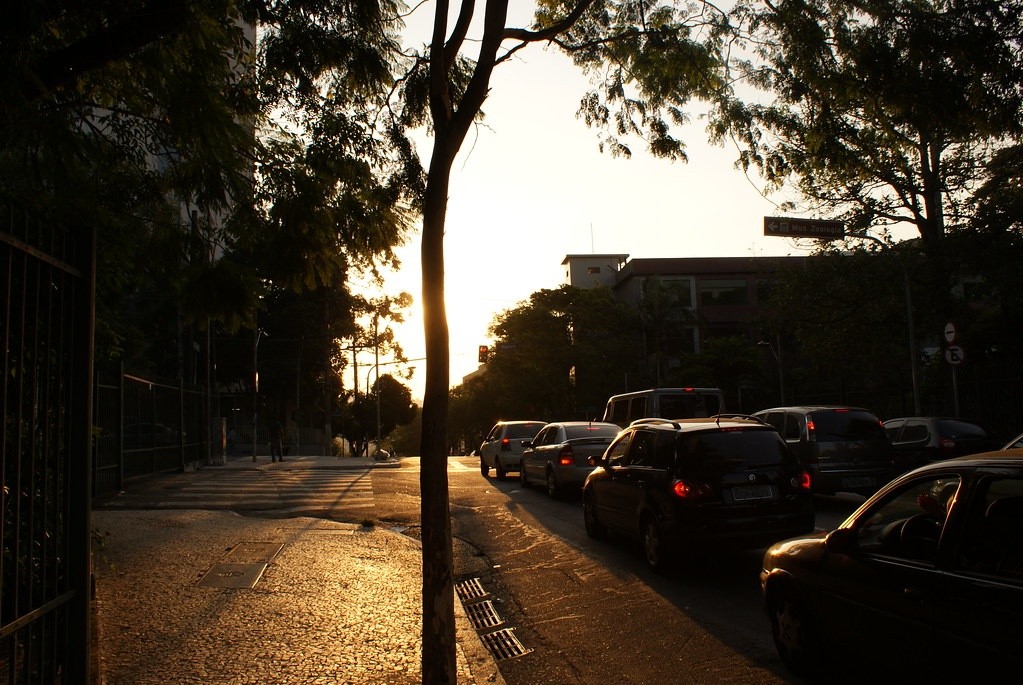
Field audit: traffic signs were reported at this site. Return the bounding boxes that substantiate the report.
[764,217,844,240]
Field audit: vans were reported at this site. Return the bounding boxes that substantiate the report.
[593,387,726,431]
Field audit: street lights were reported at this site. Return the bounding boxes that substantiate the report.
[757,335,784,407]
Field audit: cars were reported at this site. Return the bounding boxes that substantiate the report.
[761,449,1023,685]
[929,433,1023,518]
[520,422,624,503]
[882,417,990,478]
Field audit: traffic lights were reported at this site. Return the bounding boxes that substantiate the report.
[479,345,488,362]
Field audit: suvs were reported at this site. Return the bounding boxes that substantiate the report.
[479,421,549,482]
[584,413,815,585]
[745,407,897,501]
